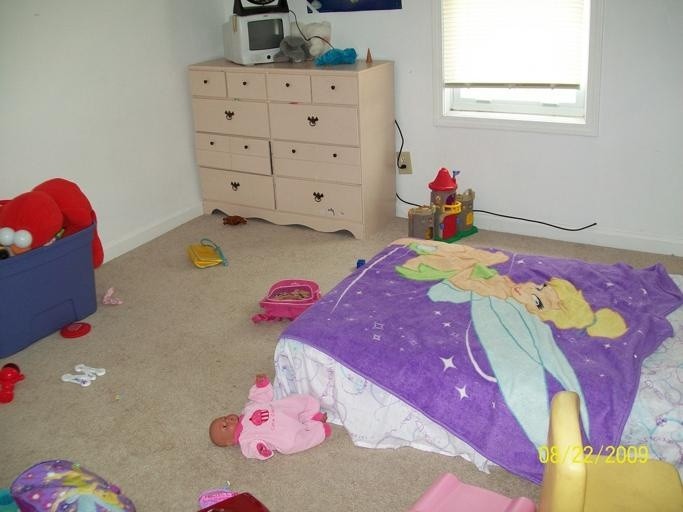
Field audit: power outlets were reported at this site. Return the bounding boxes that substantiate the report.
[396,152,412,175]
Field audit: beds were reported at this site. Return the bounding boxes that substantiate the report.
[273,236,683,468]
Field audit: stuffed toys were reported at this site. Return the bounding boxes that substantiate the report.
[0,177,104,269]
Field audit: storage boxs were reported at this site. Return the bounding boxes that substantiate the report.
[0,218,99,360]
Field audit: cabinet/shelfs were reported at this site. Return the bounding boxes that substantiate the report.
[186,60,397,242]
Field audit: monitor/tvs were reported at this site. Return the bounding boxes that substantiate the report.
[221,12,293,65]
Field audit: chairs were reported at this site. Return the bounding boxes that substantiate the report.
[540,391,682,512]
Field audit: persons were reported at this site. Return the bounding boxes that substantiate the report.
[207,371,332,462]
[384,235,630,339]
